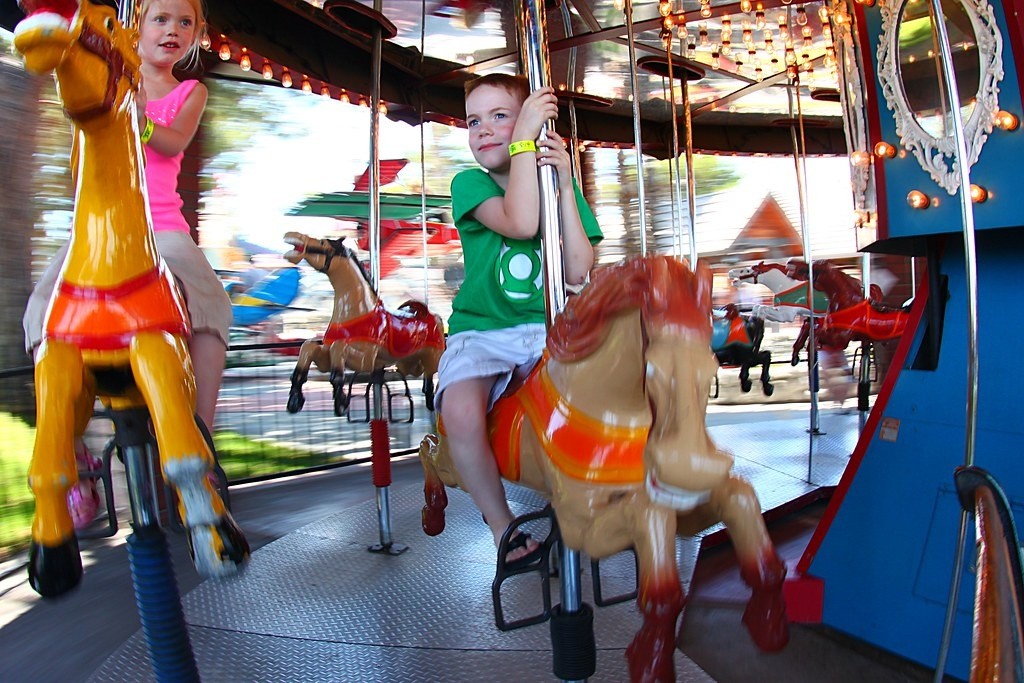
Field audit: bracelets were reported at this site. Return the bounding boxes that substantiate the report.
[508,140,537,157]
[140,115,154,144]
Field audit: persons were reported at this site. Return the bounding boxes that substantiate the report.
[431,72,605,569]
[21,0,235,534]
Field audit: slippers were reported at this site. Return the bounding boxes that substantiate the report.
[505,531,545,569]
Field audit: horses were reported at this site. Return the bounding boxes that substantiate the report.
[421,255,787,682]
[710,258,898,396]
[11,0,246,600]
[283,233,445,416]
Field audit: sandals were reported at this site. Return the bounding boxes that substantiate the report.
[66,444,103,530]
